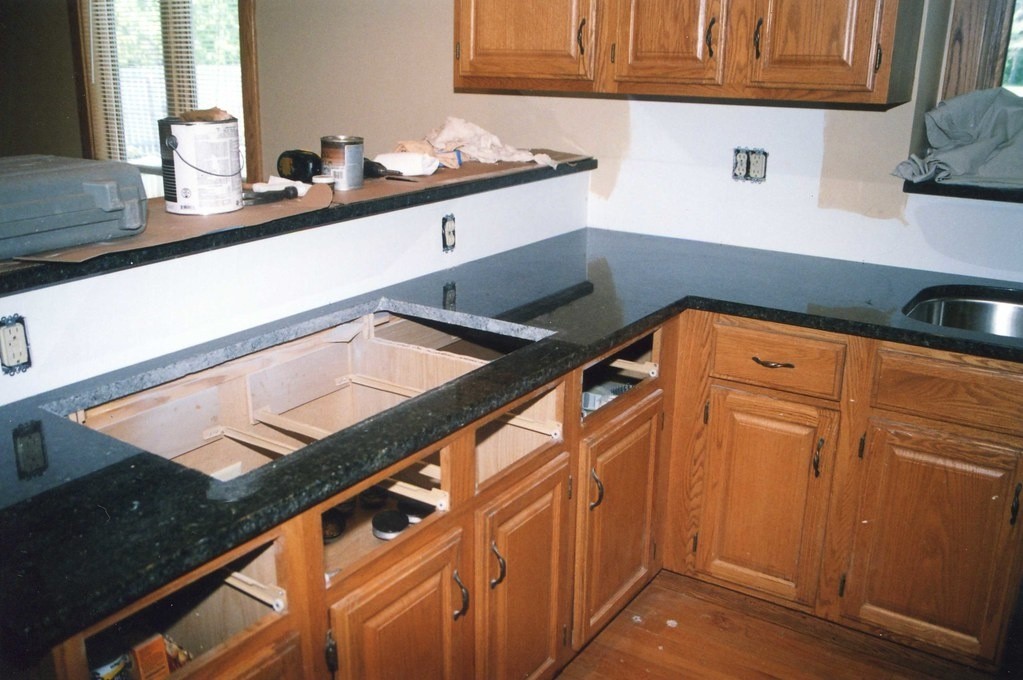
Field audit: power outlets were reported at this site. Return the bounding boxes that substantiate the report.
[12,420,49,481]
[442,281,457,306]
[732,146,768,184]
[441,213,456,253]
[0,313,33,376]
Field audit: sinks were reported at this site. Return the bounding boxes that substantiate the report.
[902,283,1023,340]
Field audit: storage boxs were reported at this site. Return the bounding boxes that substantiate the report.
[450,0,925,115]
[0,153,150,261]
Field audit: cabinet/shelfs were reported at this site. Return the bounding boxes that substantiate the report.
[38,309,1023,680]
